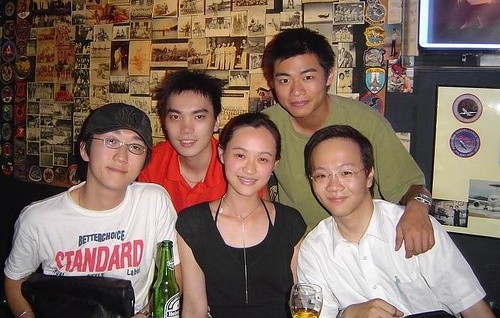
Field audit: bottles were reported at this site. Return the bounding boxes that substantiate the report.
[149,239,180,318]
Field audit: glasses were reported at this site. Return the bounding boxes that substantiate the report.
[310,164,369,184]
[87,137,147,155]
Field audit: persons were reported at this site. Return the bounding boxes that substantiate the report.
[3,102,179,318]
[297,124,495,318]
[240,38,248,69]
[236,0,267,6]
[214,38,237,69]
[71,0,232,156]
[175,111,307,318]
[255,27,435,259]
[28,23,84,165]
[249,19,264,31]
[134,68,271,214]
[339,73,346,88]
[339,47,353,68]
[230,75,249,85]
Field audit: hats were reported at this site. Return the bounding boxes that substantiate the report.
[82,103,153,149]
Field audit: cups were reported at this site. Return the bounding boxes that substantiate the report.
[289,282,323,318]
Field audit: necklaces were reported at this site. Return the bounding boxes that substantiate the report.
[222,198,264,303]
[177,155,210,183]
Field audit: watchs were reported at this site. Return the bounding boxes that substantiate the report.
[408,193,432,205]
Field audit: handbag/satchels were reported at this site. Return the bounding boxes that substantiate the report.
[22,274,136,318]
